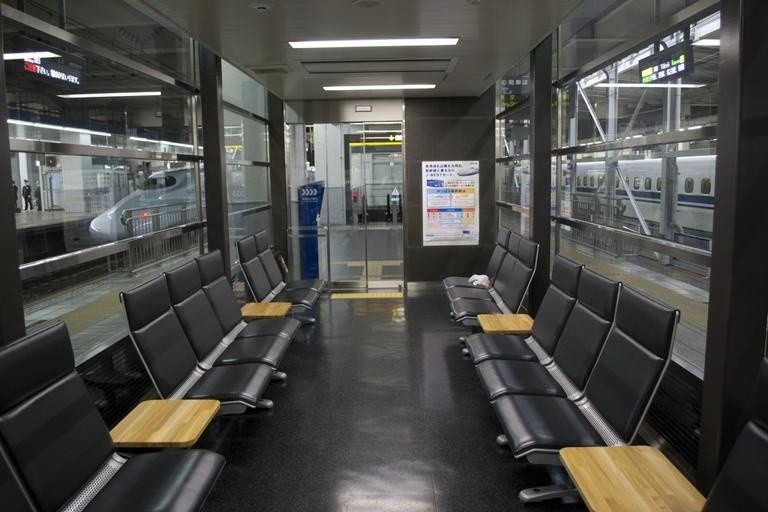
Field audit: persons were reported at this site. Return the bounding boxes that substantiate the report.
[22,180,34,211]
[12,180,19,212]
[35,180,41,211]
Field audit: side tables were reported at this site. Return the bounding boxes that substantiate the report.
[477,312,536,335]
[558,442,701,512]
[239,300,294,318]
[111,398,223,444]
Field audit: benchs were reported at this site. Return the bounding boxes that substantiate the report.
[235,231,325,324]
[442,228,538,348]
[464,255,682,500]
[120,248,304,417]
[0,318,230,512]
[706,420,767,512]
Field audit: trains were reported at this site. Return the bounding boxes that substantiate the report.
[551,155,717,240]
[88,166,207,245]
